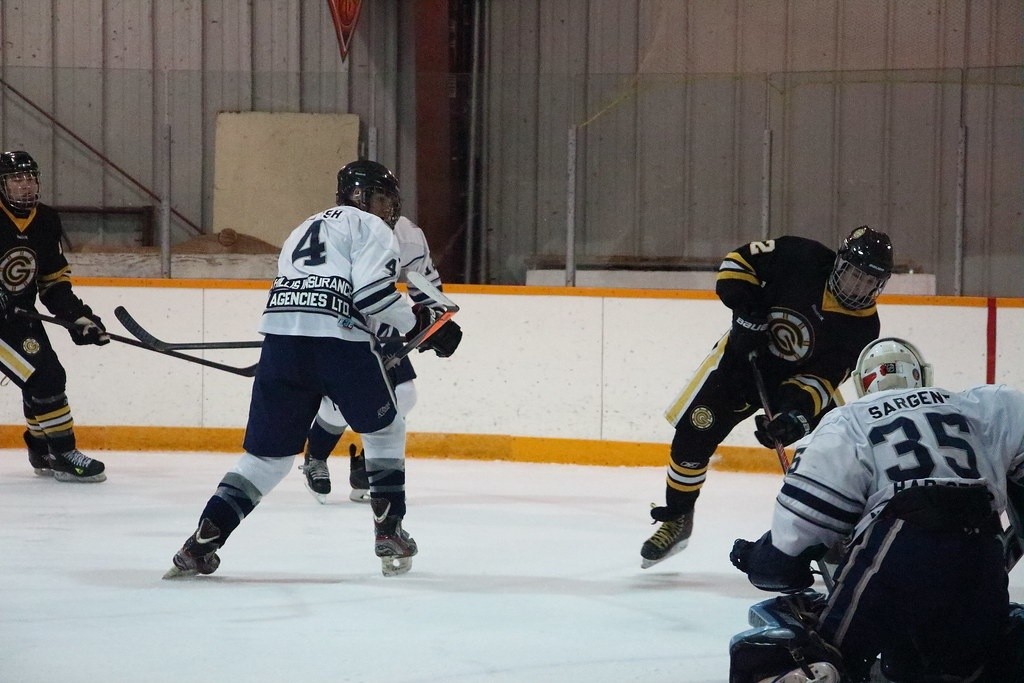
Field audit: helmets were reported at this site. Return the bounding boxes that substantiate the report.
[827,225,896,313]
[855,337,932,396]
[334,159,401,231]
[0,151,44,212]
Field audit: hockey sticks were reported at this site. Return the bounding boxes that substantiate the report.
[749,350,835,595]
[14,305,259,377]
[114,306,408,353]
[383,272,460,372]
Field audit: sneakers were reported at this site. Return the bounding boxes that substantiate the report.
[349,443,371,501]
[371,497,417,574]
[24,430,106,483]
[757,663,840,683]
[301,448,332,504]
[641,502,695,570]
[161,517,221,581]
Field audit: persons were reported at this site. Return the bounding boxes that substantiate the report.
[639,224,895,567]
[162,158,462,585]
[301,176,441,503]
[729,337,1024,682]
[0,149,109,482]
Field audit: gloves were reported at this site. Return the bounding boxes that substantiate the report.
[54,299,110,346]
[405,302,463,358]
[731,294,769,363]
[754,409,812,448]
